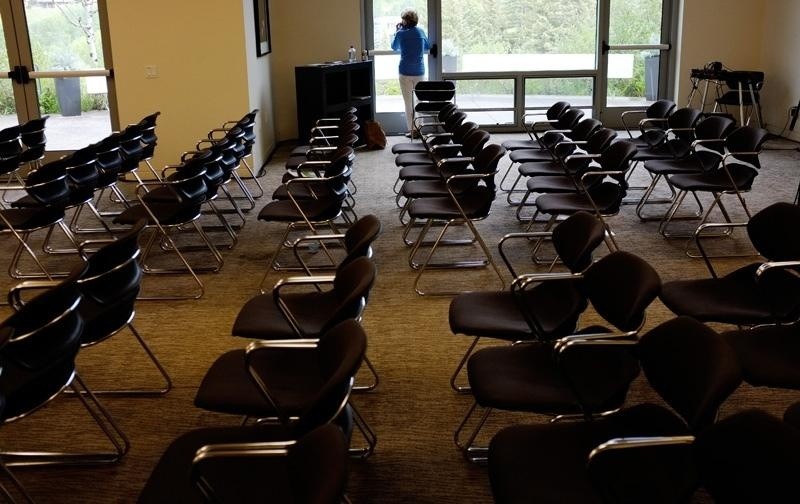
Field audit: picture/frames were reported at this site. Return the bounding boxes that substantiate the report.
[254,0,272,57]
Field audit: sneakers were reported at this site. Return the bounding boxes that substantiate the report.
[406,133,418,138]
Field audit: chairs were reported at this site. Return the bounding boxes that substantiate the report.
[655,197,796,322]
[2,70,796,303]
[4,217,176,455]
[459,312,749,494]
[219,254,382,393]
[713,261,798,396]
[434,248,666,446]
[128,423,350,504]
[185,318,375,461]
[2,293,126,503]
[569,404,799,501]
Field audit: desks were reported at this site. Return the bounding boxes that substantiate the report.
[294,61,374,151]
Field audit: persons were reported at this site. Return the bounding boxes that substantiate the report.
[391,11,430,139]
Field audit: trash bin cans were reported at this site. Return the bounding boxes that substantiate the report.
[645,55,660,101]
[54,78,82,116]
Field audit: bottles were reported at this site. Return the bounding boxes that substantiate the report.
[348,44,357,61]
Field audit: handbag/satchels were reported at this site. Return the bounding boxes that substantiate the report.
[366,119,386,149]
